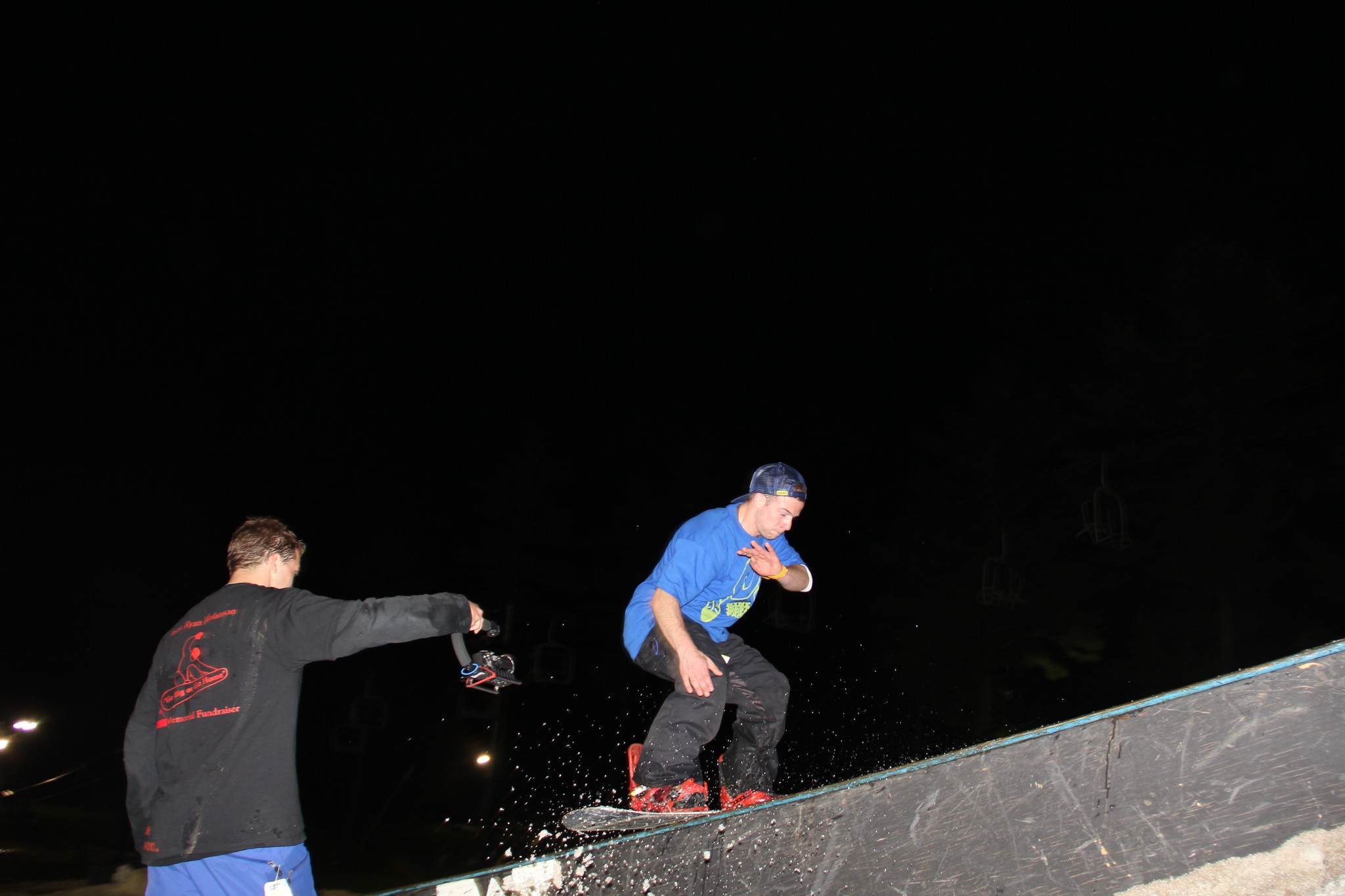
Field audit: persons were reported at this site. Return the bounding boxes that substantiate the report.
[124,518,484,896]
[622,462,813,812]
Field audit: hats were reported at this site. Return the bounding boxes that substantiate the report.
[731,461,808,504]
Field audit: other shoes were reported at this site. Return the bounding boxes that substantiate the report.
[724,792,783,811]
[635,786,705,809]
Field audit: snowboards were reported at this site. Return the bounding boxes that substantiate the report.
[562,806,725,832]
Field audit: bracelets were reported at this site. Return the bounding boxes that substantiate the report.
[768,566,788,579]
